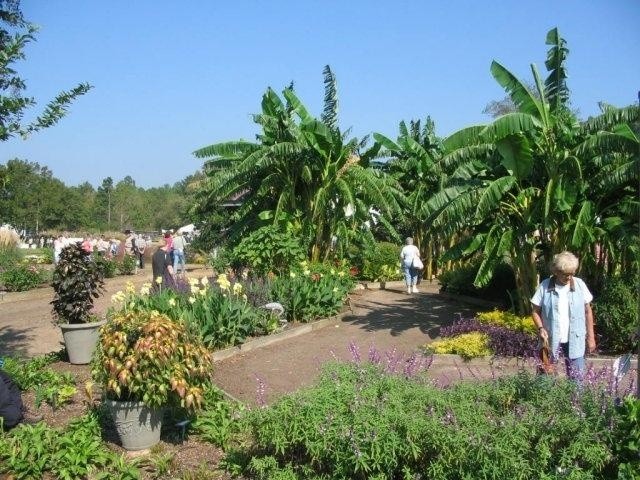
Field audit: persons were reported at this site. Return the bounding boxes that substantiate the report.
[152,240,175,289]
[399,236,423,293]
[164,227,191,274]
[25,228,147,275]
[528,250,596,383]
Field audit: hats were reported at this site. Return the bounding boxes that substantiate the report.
[124,230,132,234]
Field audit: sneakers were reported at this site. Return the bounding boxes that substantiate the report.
[412,288,419,293]
[407,290,411,294]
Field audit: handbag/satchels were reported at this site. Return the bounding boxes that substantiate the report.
[536,347,555,375]
[412,256,423,270]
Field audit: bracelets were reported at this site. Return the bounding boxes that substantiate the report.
[537,325,542,331]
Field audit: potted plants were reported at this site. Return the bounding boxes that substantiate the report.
[47,244,106,366]
[80,303,216,455]
